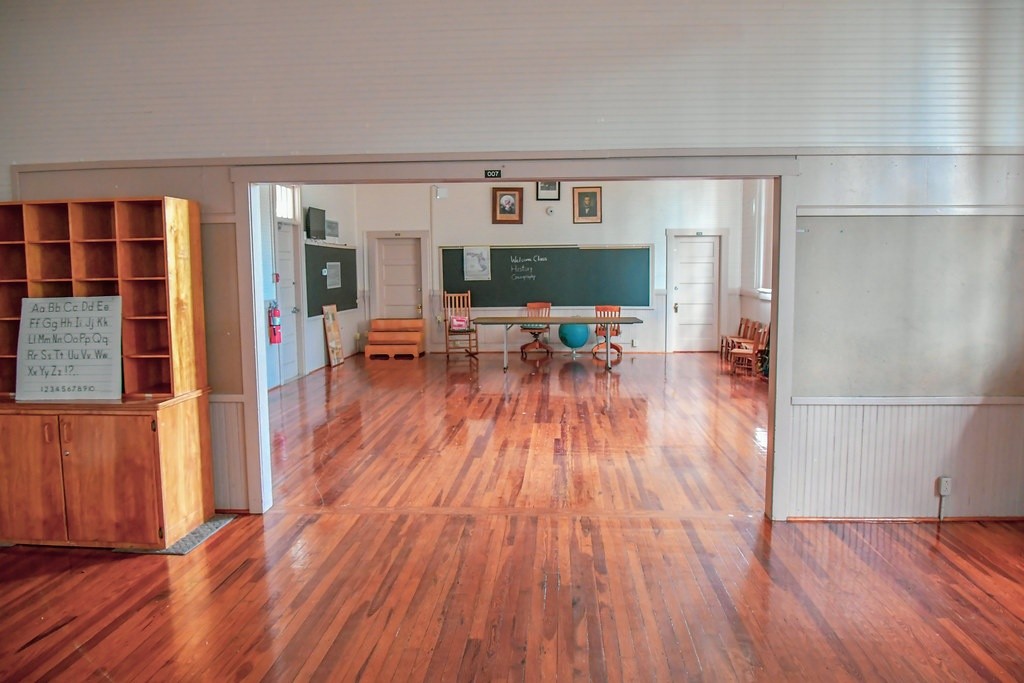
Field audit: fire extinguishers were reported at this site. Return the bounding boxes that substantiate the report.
[268,300,282,344]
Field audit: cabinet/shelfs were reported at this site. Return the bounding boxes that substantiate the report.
[0,197,207,401]
[0,392,214,548]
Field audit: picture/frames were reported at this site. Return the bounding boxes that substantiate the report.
[572,186,603,223]
[536,181,561,202]
[492,187,523,224]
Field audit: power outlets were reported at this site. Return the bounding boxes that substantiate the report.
[940,477,952,496]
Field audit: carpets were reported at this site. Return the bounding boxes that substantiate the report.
[112,514,239,556]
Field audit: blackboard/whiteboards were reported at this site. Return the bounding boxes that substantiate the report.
[438,243,655,310]
[305,240,359,319]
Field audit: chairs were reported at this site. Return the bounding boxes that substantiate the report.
[443,291,479,363]
[519,302,554,359]
[592,305,624,358]
[720,318,770,375]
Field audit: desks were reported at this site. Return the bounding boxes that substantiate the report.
[473,317,642,371]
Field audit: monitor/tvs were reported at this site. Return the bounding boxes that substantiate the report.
[307,207,326,239]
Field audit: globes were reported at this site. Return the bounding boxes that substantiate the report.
[558,357,591,395]
[558,315,591,357]
